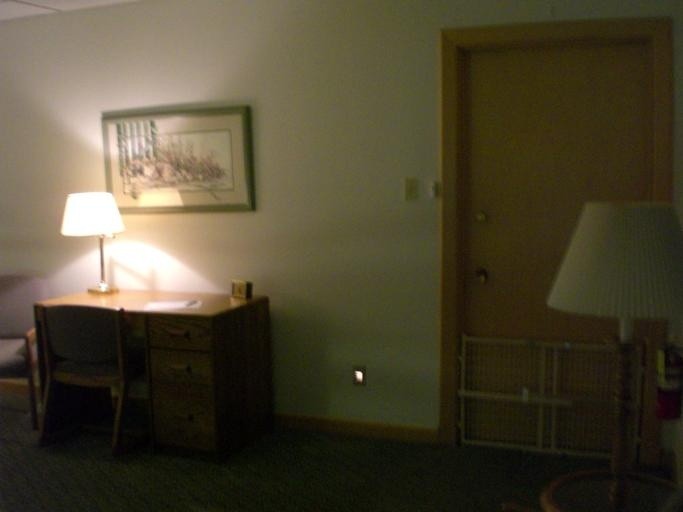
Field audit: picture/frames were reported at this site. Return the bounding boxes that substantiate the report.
[100,103,257,215]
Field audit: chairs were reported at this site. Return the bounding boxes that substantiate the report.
[32,301,147,457]
[1,274,47,435]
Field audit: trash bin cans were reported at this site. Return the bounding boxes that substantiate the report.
[657,332,683,421]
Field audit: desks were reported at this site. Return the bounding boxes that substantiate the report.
[31,290,276,467]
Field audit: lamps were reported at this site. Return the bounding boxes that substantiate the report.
[59,191,126,294]
[542,200,681,512]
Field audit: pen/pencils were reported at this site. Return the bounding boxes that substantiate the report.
[185,299,197,307]
